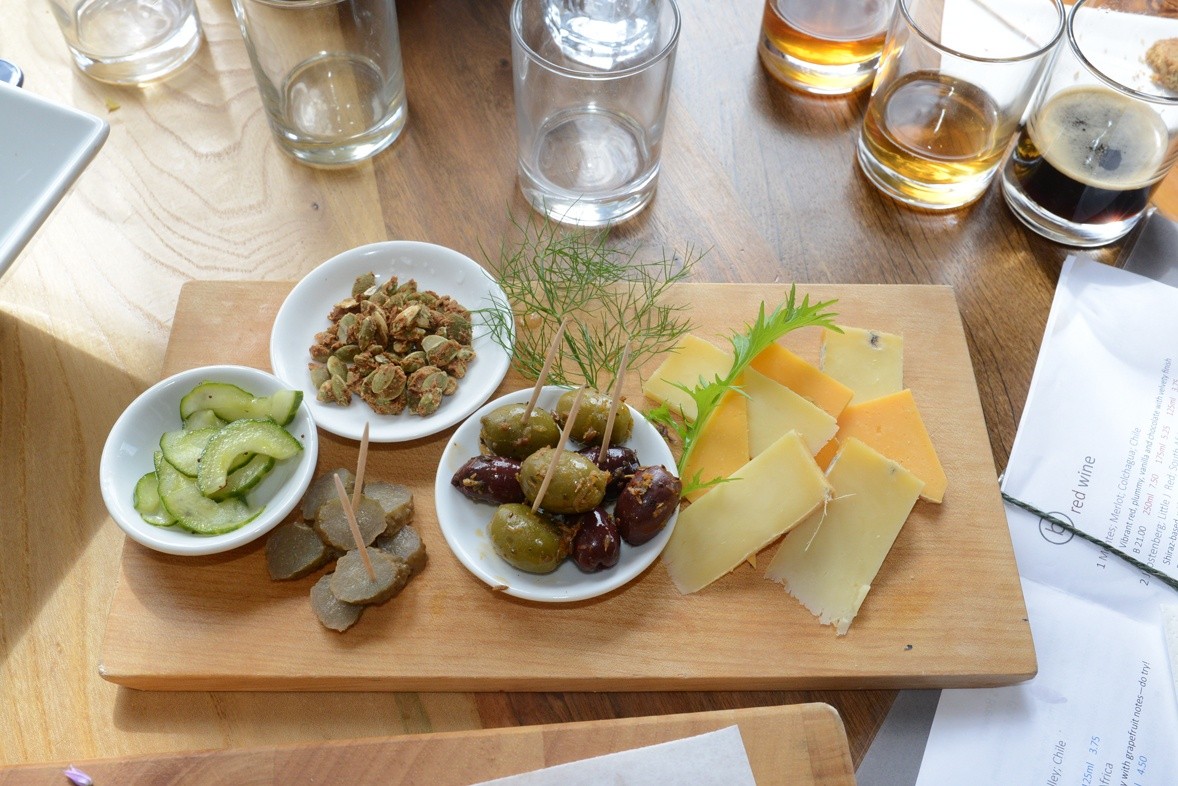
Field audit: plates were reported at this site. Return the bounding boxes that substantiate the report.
[0,81,111,273]
[434,384,680,603]
[268,240,516,443]
[97,365,319,557]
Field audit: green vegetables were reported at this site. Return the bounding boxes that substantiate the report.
[455,196,844,504]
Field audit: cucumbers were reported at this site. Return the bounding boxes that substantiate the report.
[133,383,304,534]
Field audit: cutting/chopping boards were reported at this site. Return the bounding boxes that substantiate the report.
[92,283,1040,694]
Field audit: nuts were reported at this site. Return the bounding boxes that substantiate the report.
[306,269,473,416]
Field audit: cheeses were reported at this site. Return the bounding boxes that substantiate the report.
[643,326,947,636]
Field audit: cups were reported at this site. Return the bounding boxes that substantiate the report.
[857,0,1067,209]
[229,0,409,169]
[1000,0,1178,248]
[756,0,908,96]
[510,0,682,231]
[45,0,202,85]
